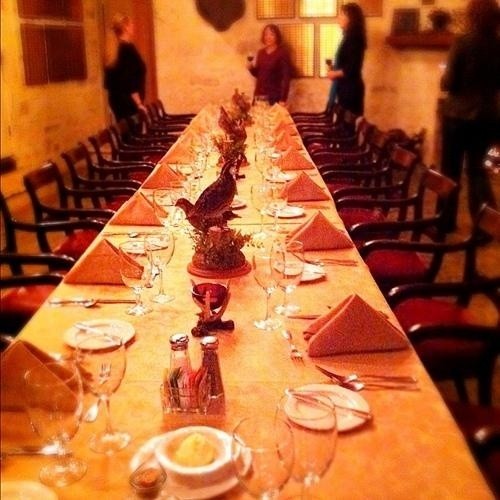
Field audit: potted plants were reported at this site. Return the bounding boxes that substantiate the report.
[391,8,420,36]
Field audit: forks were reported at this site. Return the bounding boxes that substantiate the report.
[314,364,421,392]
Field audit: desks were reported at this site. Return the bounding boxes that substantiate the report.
[3,101,497,499]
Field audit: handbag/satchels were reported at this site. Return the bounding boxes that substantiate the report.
[136,103,142,107]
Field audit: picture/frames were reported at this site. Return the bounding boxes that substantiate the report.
[391,8,420,36]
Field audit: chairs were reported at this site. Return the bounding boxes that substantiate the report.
[0,90,195,330]
[292,100,499,462]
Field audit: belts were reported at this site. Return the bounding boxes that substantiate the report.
[1,94,369,500]
[438,60,448,74]
[248,56,254,63]
[325,59,333,70]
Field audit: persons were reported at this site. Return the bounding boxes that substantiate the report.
[247,24,291,107]
[326,3,367,116]
[434,0,499,246]
[102,12,147,142]
